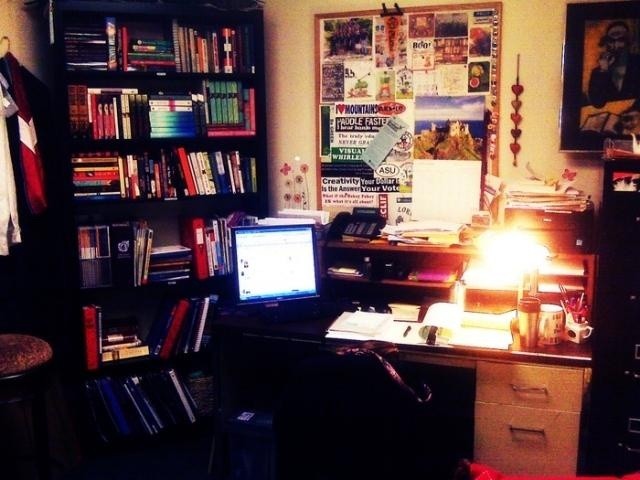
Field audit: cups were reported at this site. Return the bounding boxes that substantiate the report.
[519,297,593,348]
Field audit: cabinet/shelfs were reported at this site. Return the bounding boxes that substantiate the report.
[24,0,269,451]
[321,239,599,304]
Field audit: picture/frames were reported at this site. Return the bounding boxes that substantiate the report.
[557,1,640,153]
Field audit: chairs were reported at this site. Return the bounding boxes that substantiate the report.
[280,347,438,477]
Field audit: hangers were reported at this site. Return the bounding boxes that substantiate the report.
[0,35,10,56]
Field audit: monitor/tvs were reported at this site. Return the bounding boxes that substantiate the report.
[231,224,322,309]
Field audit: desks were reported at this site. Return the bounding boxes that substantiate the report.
[194,293,595,476]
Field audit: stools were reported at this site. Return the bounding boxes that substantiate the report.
[0,333,54,479]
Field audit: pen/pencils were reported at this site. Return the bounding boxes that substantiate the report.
[403,326,411,337]
[559,282,588,323]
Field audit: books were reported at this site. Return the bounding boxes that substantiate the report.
[258,208,330,230]
[70,149,257,434]
[65,16,257,140]
[329,311,388,336]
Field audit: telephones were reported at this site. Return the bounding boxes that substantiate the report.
[327,207,386,240]
